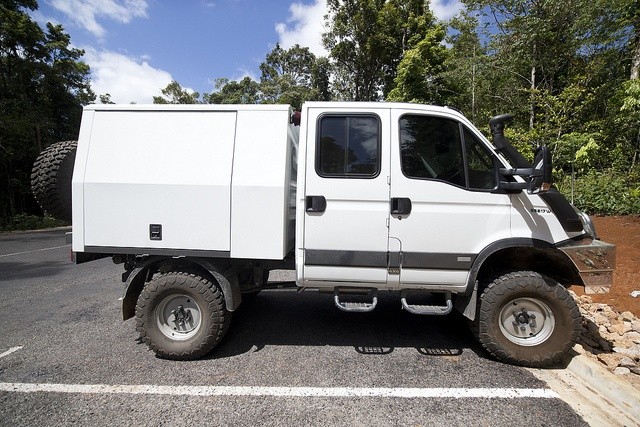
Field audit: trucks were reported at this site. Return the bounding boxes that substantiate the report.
[29,100,615,368]
